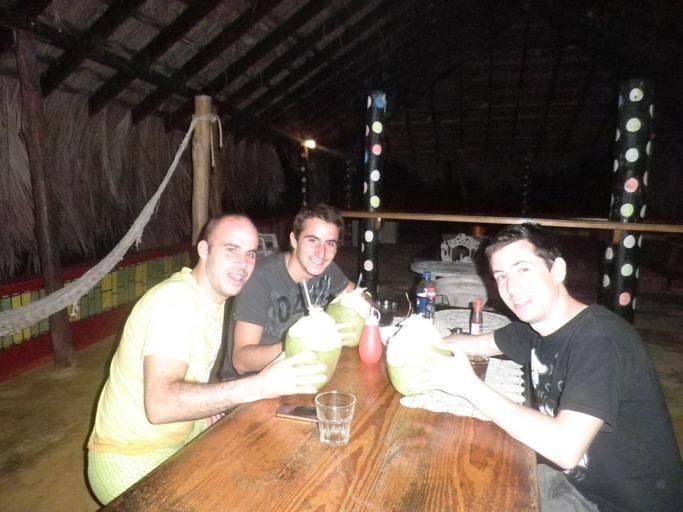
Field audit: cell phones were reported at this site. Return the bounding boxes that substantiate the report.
[276,403,318,420]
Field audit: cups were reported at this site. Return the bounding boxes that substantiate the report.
[315,392,357,447]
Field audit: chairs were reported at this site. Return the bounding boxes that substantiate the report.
[429,233,490,308]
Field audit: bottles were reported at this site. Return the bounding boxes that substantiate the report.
[471,297,483,334]
[359,306,383,364]
[417,271,436,321]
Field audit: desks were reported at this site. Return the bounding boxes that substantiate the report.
[409,258,483,279]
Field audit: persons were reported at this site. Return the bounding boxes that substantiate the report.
[418,222,683,511]
[218,204,375,416]
[86,210,328,507]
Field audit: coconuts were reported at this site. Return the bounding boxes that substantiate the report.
[285,306,341,390]
[326,287,370,347]
[385,313,443,394]
